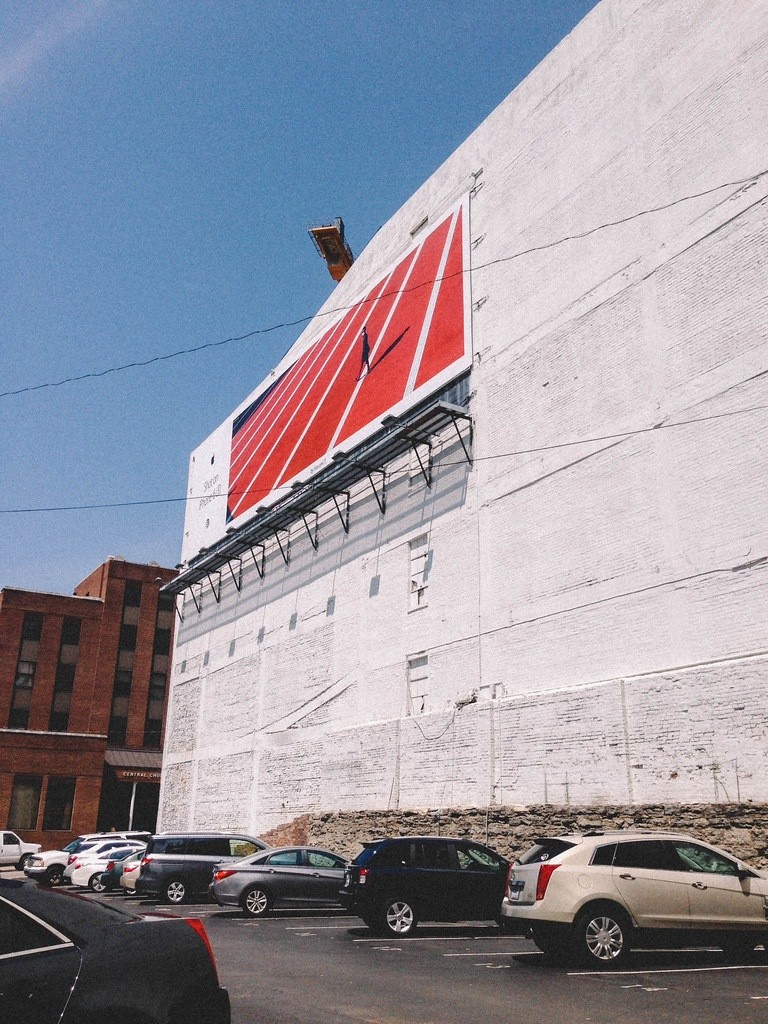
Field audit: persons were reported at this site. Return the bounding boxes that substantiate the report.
[354,325,372,381]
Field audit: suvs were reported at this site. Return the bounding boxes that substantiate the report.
[499,828,767,968]
[135,831,275,904]
[0,830,43,871]
[337,834,511,936]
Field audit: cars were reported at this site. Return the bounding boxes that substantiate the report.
[210,844,351,918]
[0,877,232,1024]
[24,830,152,894]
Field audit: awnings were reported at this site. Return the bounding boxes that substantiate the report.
[101,746,163,783]
